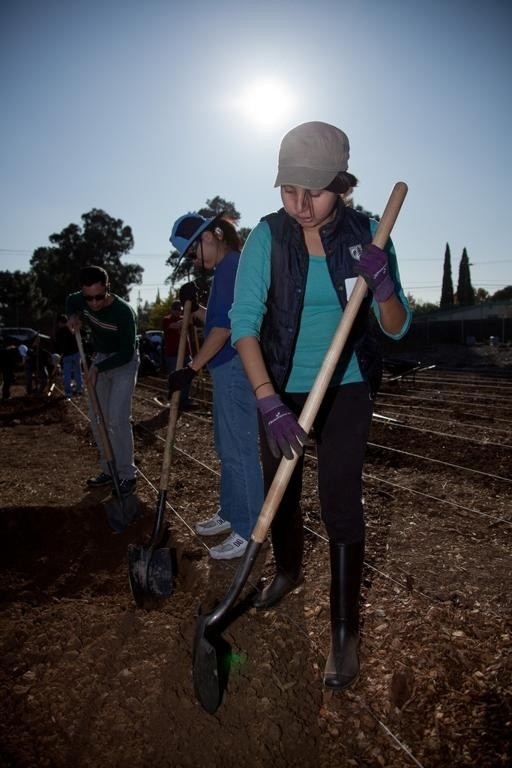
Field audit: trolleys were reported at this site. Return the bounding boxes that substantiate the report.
[381,357,438,385]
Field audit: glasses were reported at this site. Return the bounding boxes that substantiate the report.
[81,294,106,301]
[186,242,199,261]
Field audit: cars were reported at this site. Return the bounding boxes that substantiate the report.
[136,329,166,353]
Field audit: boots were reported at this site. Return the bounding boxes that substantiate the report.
[322,541,365,690]
[249,519,305,607]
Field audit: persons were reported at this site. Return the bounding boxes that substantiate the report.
[0,314,163,403]
[167,210,270,564]
[153,300,199,410]
[64,266,143,496]
[224,119,414,692]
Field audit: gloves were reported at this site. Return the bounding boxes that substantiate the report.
[166,365,197,399]
[257,395,313,459]
[179,281,200,313]
[352,242,399,302]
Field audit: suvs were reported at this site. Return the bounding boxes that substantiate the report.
[2,326,53,351]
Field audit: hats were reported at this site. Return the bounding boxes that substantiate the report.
[274,120,350,191]
[168,213,219,263]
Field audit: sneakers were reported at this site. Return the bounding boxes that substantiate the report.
[87,473,114,486]
[209,531,249,560]
[195,512,233,536]
[112,478,137,498]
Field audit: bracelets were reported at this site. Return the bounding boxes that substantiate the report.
[253,381,274,398]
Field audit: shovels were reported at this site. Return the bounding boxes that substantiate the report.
[193,182,408,715]
[127,301,191,613]
[74,326,138,532]
